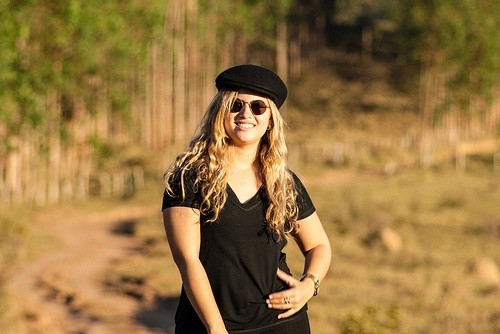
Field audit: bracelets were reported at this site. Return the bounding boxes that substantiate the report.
[161,64,332,334]
[300,272,321,299]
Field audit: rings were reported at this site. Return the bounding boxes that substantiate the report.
[284,297,290,304]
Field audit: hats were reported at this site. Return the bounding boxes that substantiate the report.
[215,64,288,110]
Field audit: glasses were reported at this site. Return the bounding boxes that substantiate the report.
[229,97,271,115]
